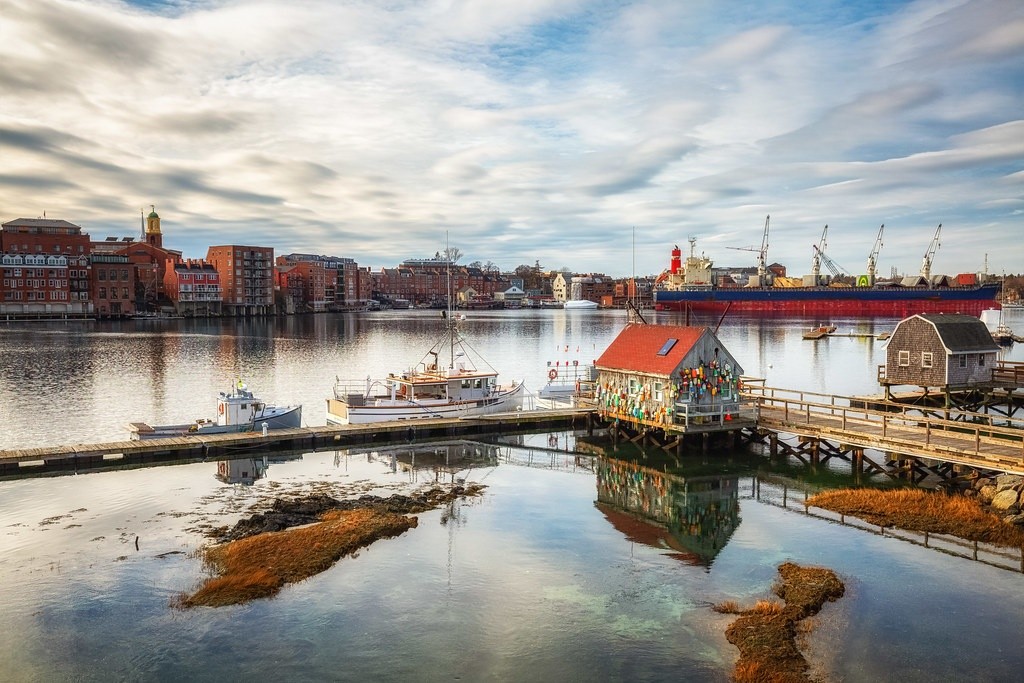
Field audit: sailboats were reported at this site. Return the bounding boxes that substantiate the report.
[991,268,1012,346]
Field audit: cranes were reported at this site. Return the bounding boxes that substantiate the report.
[920,222,942,279]
[811,224,885,287]
[726,214,770,284]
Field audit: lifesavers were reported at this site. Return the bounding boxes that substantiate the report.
[549,369,558,379]
[401,385,406,395]
[219,403,224,415]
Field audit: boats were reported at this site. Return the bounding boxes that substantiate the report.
[120,378,302,441]
[326,230,524,427]
[564,300,598,309]
